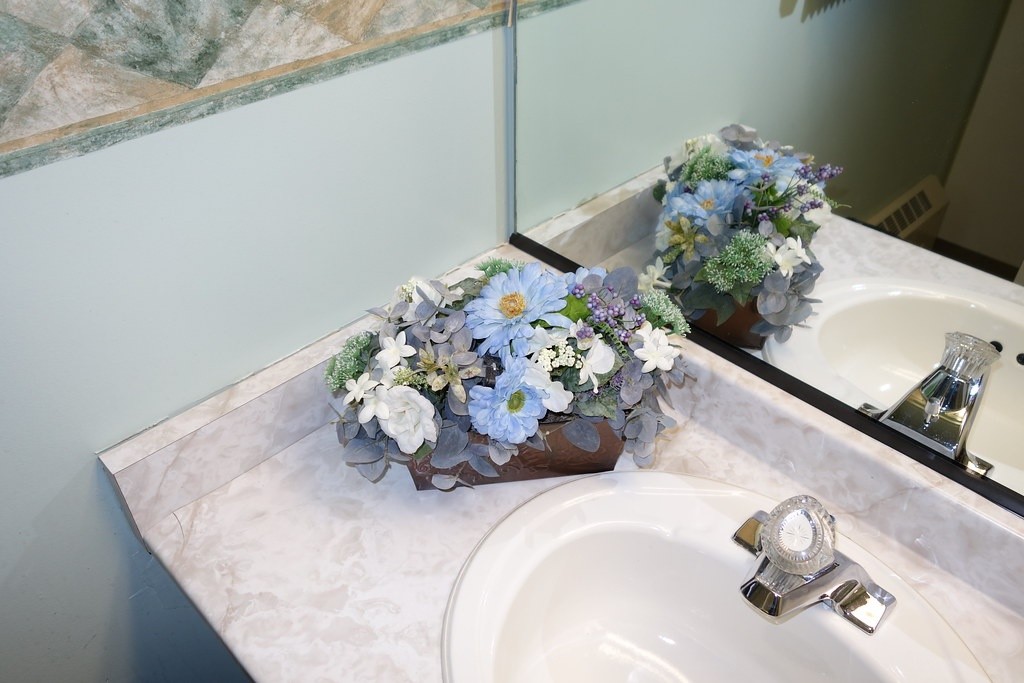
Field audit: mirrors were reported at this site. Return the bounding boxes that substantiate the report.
[503,1,1023,521]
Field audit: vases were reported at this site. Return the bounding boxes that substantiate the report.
[689,289,770,350]
[405,412,626,492]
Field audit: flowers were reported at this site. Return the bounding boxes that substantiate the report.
[650,121,854,338]
[325,255,692,490]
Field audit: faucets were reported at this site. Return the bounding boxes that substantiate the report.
[857,332,994,478]
[732,496,897,635]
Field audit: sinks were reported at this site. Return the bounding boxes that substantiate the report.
[760,275,1024,494]
[440,471,992,683]
